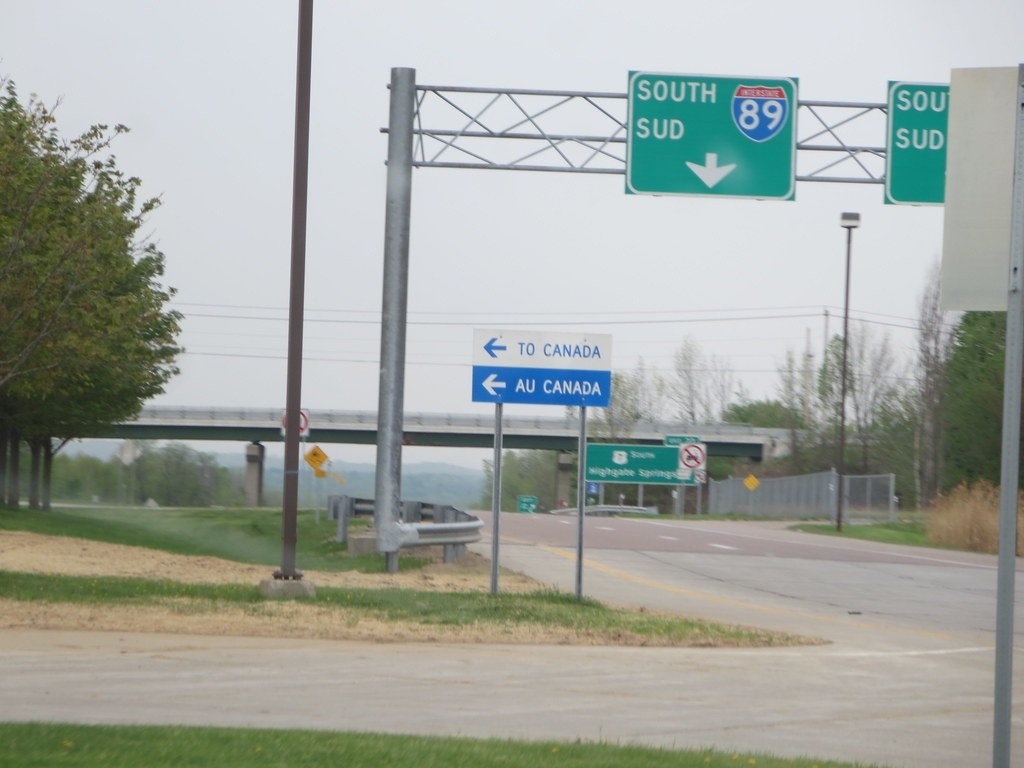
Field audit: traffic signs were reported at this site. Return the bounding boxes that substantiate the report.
[624,69,800,204]
[469,330,614,408]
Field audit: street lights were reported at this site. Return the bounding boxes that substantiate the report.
[836,210,860,530]
